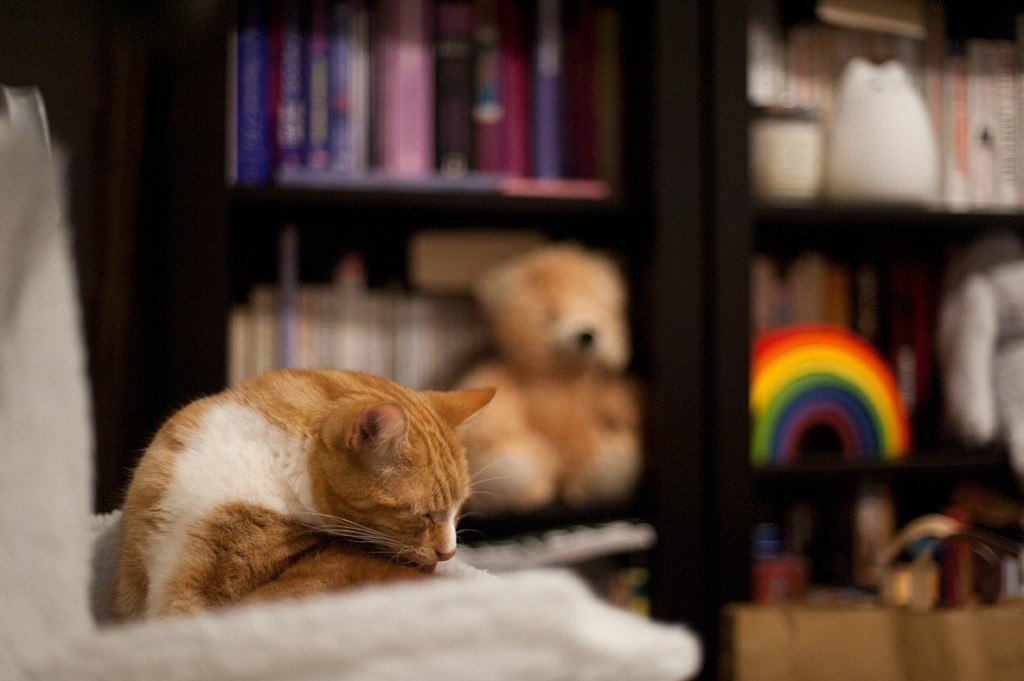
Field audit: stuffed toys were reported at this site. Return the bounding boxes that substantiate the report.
[445,238,644,514]
[934,257,1024,477]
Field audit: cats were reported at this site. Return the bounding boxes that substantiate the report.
[106,366,496,621]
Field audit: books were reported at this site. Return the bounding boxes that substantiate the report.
[227,225,547,393]
[227,0,627,199]
[744,0,1024,215]
[747,240,1024,610]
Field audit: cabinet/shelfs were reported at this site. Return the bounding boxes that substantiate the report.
[80,0,712,681]
[701,0,1024,681]
